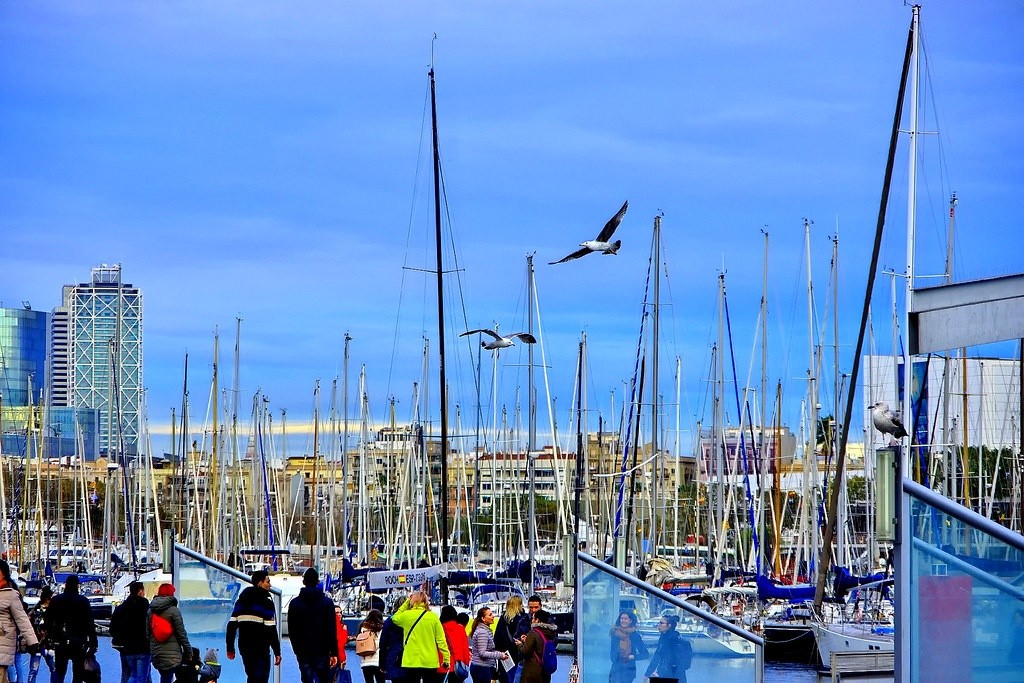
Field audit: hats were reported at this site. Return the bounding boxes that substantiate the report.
[204,647,219,663]
[158,583,175,596]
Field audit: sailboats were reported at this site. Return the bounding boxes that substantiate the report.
[2,2,1022,683]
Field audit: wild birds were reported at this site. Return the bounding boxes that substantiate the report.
[460,329,537,359]
[868,403,909,448]
[547,200,630,266]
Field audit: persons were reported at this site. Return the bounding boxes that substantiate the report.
[145,583,192,683]
[195,648,222,683]
[178,646,217,683]
[44,575,98,683]
[109,599,131,683]
[288,567,339,683]
[8,650,25,682]
[120,581,153,683]
[493,594,558,683]
[607,608,650,683]
[0,559,40,683]
[379,595,407,683]
[471,607,509,683]
[642,614,688,683]
[226,570,281,683]
[334,606,349,682]
[437,605,471,683]
[358,610,384,683]
[393,590,451,683]
[17,590,56,683]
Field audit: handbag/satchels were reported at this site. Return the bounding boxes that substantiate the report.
[395,647,404,668]
[334,667,352,683]
[83,648,102,683]
[454,659,469,679]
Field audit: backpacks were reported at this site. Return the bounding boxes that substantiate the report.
[151,611,173,643]
[354,629,379,660]
[671,631,693,670]
[532,628,557,674]
